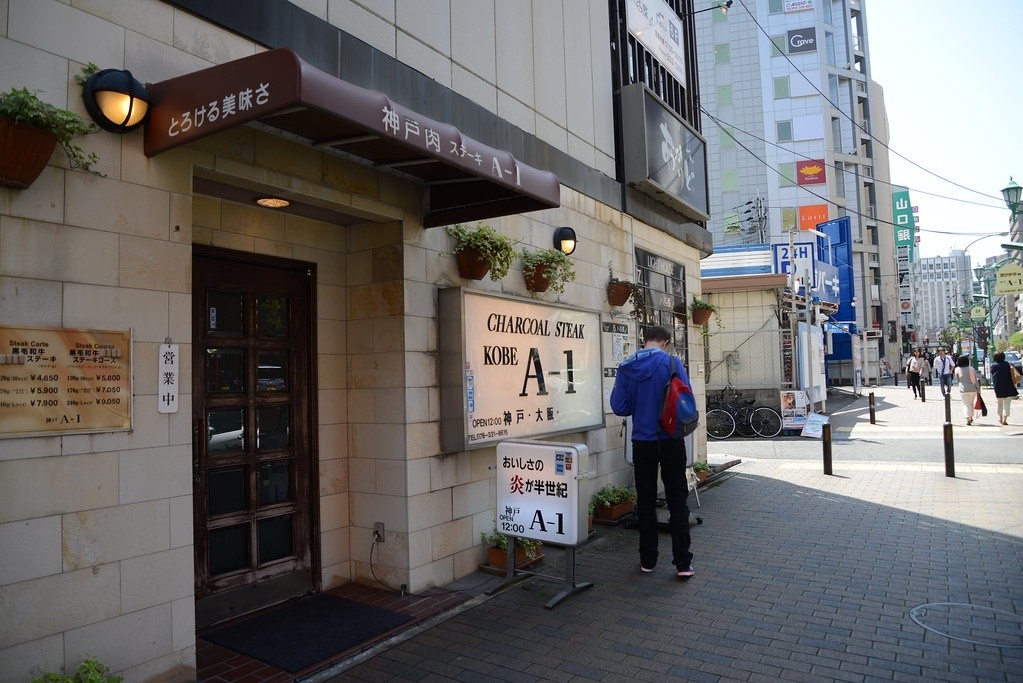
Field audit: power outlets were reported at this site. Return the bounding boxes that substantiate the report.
[374,522,384,542]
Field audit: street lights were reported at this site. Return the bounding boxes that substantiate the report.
[962,232,1011,368]
[955,275,984,318]
[972,261,1001,354]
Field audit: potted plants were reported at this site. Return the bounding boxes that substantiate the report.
[480,518,544,574]
[608,278,648,319]
[518,245,576,294]
[686,293,725,337]
[693,462,713,481]
[439,222,525,282]
[588,483,638,530]
[0,62,107,190]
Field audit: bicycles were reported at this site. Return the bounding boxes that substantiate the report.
[706,386,783,441]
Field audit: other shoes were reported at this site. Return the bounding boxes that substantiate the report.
[966,419,973,425]
[1003,422,1008,425]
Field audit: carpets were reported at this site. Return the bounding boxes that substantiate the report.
[199,595,416,674]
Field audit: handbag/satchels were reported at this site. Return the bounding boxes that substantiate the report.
[975,393,987,416]
[1010,362,1021,384]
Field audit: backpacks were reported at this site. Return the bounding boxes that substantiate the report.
[659,354,699,437]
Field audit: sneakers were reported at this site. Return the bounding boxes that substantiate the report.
[641,565,653,572]
[678,565,694,575]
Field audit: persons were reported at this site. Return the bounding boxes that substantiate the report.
[609,326,693,575]
[906,347,973,400]
[990,351,1020,425]
[955,356,979,425]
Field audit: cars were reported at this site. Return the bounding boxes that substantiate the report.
[976,349,985,365]
[961,350,970,357]
[1001,350,1023,375]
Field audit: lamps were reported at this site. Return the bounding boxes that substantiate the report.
[553,226,576,255]
[83,69,151,134]
[680,0,733,20]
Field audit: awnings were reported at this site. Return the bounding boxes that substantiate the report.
[143,48,560,228]
